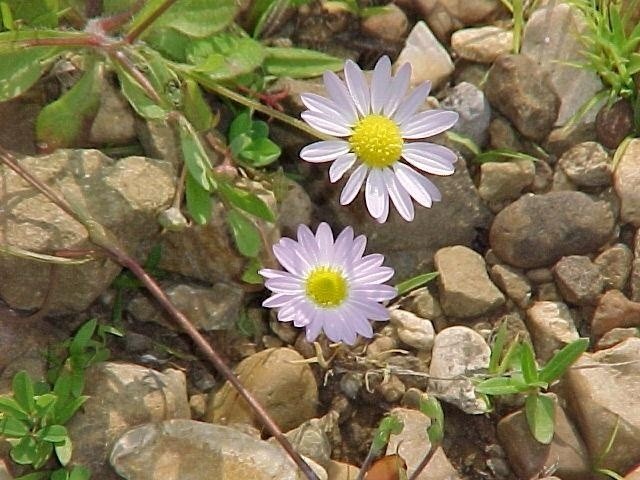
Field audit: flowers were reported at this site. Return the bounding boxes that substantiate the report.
[254,215,405,357]
[280,52,458,227]
